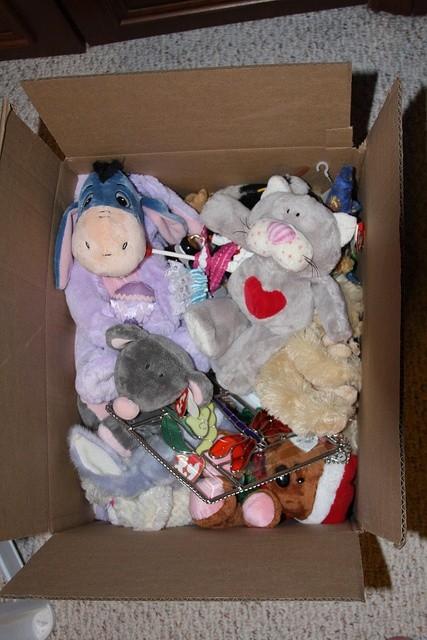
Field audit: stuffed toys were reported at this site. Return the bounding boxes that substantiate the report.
[186,187,211,215]
[59,174,212,406]
[52,160,205,291]
[253,275,365,438]
[186,435,360,530]
[182,174,352,397]
[66,424,187,498]
[77,322,216,450]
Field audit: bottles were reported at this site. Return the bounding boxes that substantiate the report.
[327,165,356,212]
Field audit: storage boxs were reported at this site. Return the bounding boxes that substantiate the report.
[0,61,407,601]
